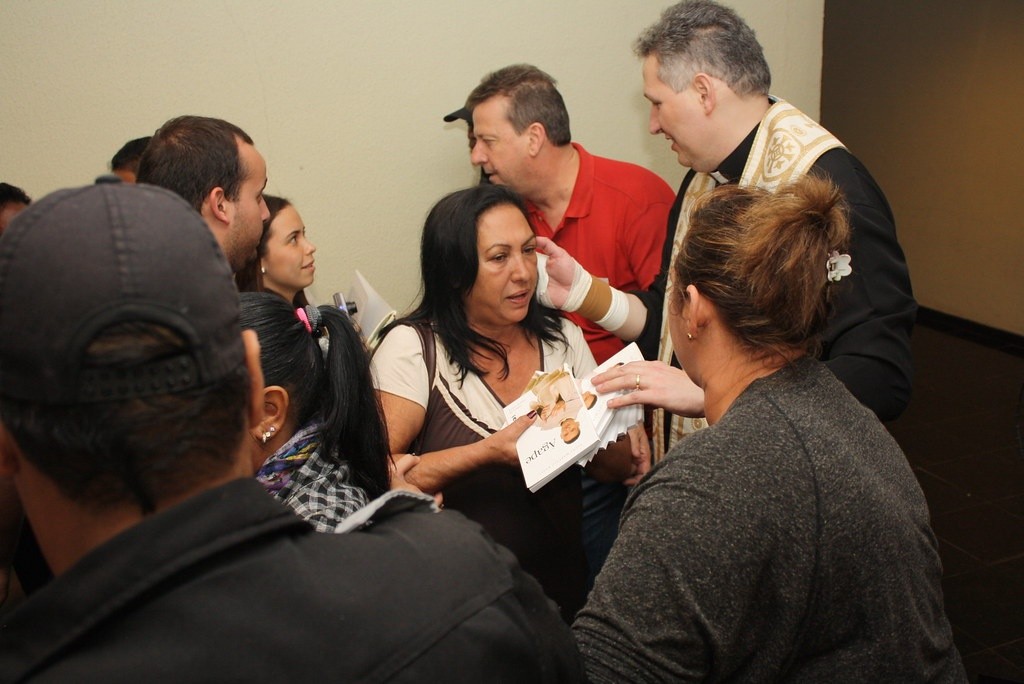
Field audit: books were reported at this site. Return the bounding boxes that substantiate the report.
[499,339,645,494]
[347,269,397,352]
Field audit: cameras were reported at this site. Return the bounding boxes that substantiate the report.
[333,292,358,320]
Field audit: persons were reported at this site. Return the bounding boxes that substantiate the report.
[464,63,675,581]
[575,379,598,410]
[236,287,395,537]
[367,183,649,611]
[531,374,584,443]
[0,182,33,234]
[134,114,269,274]
[444,106,492,188]
[229,191,358,359]
[530,0,921,468]
[112,138,154,185]
[571,173,971,683]
[0,177,589,684]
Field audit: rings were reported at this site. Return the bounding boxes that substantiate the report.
[636,374,641,389]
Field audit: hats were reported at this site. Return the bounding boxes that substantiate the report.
[0,174,243,405]
[444,107,473,123]
